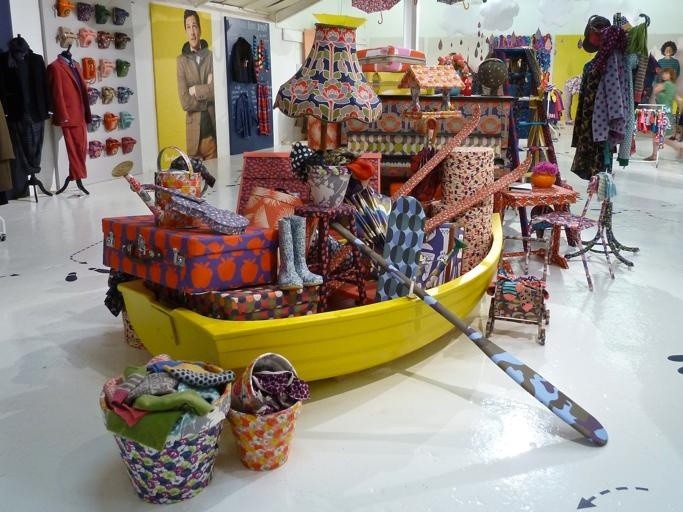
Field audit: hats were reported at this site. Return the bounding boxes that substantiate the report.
[582,16,611,54]
[477,58,508,88]
[660,40,677,56]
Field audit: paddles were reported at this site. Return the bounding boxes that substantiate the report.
[329,219,608,444]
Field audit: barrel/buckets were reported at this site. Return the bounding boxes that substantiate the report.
[231,353,298,409]
[101,361,234,506]
[227,401,302,471]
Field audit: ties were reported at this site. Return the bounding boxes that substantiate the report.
[70,63,81,88]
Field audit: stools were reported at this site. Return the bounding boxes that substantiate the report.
[293,204,368,313]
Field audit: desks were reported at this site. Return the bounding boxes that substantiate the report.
[494,184,576,271]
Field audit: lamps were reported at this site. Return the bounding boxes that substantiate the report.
[273,24,382,152]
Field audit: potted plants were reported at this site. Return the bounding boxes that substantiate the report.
[306,151,360,210]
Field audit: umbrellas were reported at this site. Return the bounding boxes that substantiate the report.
[341,184,392,254]
[436,0,487,10]
[351,0,408,25]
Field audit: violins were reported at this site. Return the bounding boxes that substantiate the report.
[130,183,249,235]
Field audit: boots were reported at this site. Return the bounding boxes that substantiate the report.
[277,215,324,291]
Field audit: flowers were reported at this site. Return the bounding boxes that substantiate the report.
[530,161,559,177]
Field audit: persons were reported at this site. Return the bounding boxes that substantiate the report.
[0,34,48,176]
[47,48,93,180]
[642,68,677,161]
[657,41,681,89]
[563,74,584,125]
[176,8,217,162]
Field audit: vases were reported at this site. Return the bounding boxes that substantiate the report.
[530,174,556,188]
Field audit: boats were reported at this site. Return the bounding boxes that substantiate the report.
[115,213,502,390]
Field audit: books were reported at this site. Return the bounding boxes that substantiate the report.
[508,182,532,193]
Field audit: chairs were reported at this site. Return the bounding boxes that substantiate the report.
[524,174,616,292]
